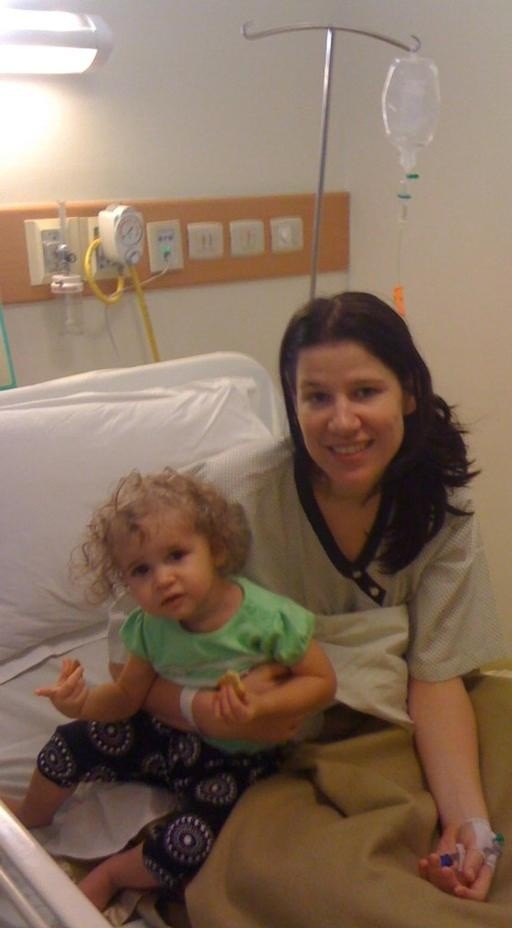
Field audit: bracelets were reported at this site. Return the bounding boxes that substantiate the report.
[180,687,200,736]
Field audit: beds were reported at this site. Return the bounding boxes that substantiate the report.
[0,351,512,928]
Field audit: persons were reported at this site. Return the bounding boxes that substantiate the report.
[1,466,337,914]
[107,291,512,901]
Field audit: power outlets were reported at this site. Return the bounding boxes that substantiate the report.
[24,218,81,286]
[86,214,137,279]
[146,219,182,273]
[269,215,303,253]
[187,222,224,259]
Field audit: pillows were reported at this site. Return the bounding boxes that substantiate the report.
[0,379,273,684]
